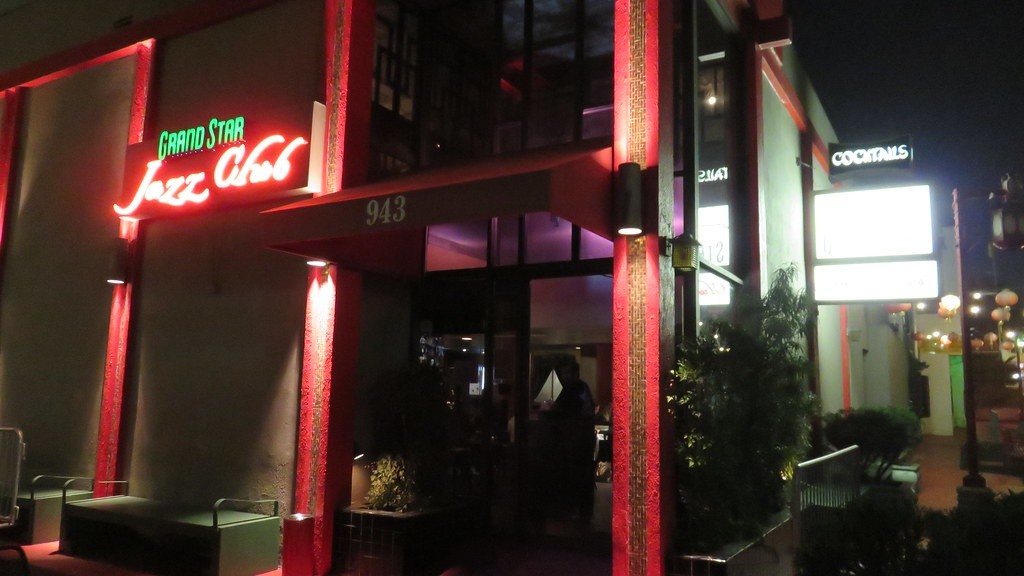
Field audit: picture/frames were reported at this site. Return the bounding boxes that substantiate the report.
[528,348,583,412]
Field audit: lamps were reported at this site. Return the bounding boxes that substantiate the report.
[659,227,702,274]
[107,236,130,285]
[617,161,649,237]
[352,442,365,461]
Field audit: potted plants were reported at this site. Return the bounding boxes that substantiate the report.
[332,431,490,576]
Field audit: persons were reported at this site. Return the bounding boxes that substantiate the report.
[538,362,599,522]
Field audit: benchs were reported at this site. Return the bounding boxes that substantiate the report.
[57,479,280,576]
[0,474,94,544]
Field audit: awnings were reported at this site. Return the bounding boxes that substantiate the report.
[258,141,611,271]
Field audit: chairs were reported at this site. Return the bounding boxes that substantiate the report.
[0,427,32,576]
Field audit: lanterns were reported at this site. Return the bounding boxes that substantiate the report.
[886,288,1018,353]
